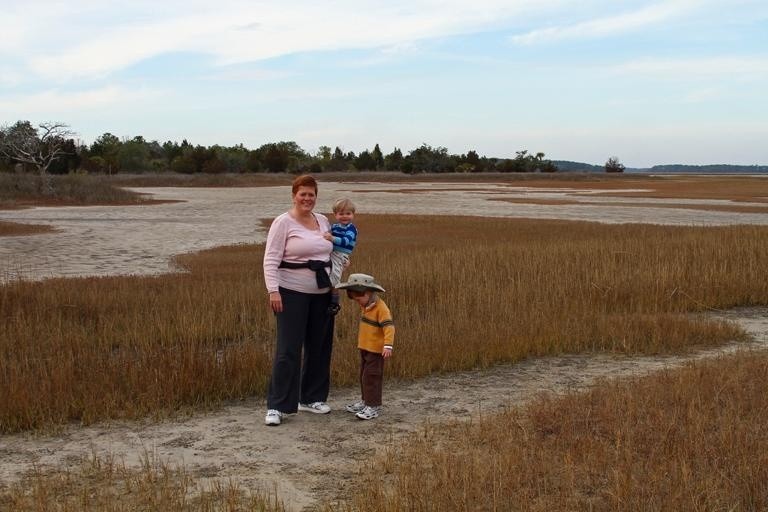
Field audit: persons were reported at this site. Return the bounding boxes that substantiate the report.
[323,197,357,315]
[261,174,352,426]
[332,273,396,419]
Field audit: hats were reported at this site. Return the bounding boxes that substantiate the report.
[335,273,386,294]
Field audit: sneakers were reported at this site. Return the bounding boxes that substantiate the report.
[329,304,341,314]
[298,402,330,415]
[346,400,364,411]
[266,410,282,426]
[355,406,379,420]
